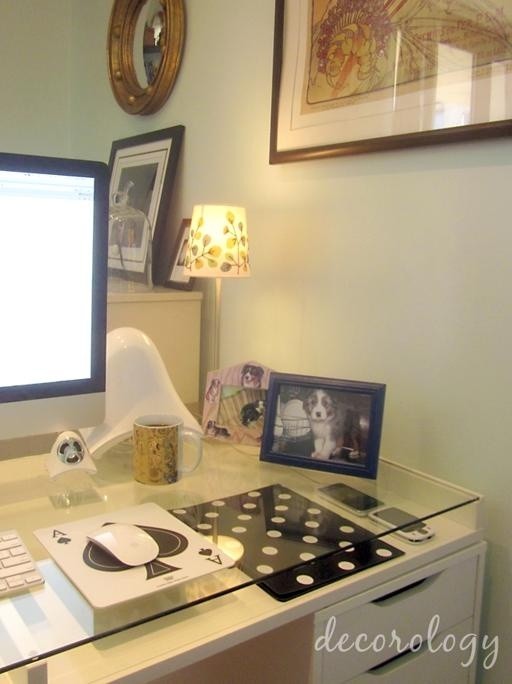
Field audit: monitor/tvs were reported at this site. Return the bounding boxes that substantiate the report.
[0,152,112,440]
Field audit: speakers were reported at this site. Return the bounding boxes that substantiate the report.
[48,431,98,479]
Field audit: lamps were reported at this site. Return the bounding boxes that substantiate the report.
[184,203,250,371]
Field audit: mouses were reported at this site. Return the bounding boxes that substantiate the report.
[86,523,160,568]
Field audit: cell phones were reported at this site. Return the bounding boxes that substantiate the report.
[368,505,435,542]
[319,482,385,517]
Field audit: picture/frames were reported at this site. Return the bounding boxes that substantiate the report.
[107,0,186,114]
[107,124,195,291]
[200,356,382,479]
[267,0,511,162]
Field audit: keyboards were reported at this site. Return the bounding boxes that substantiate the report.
[0,528,46,596]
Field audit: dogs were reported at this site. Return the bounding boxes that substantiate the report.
[240,398,266,426]
[302,388,371,462]
[240,363,264,388]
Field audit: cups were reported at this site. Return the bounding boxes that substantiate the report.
[132,413,203,485]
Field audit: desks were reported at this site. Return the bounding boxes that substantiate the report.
[0,446,490,683]
